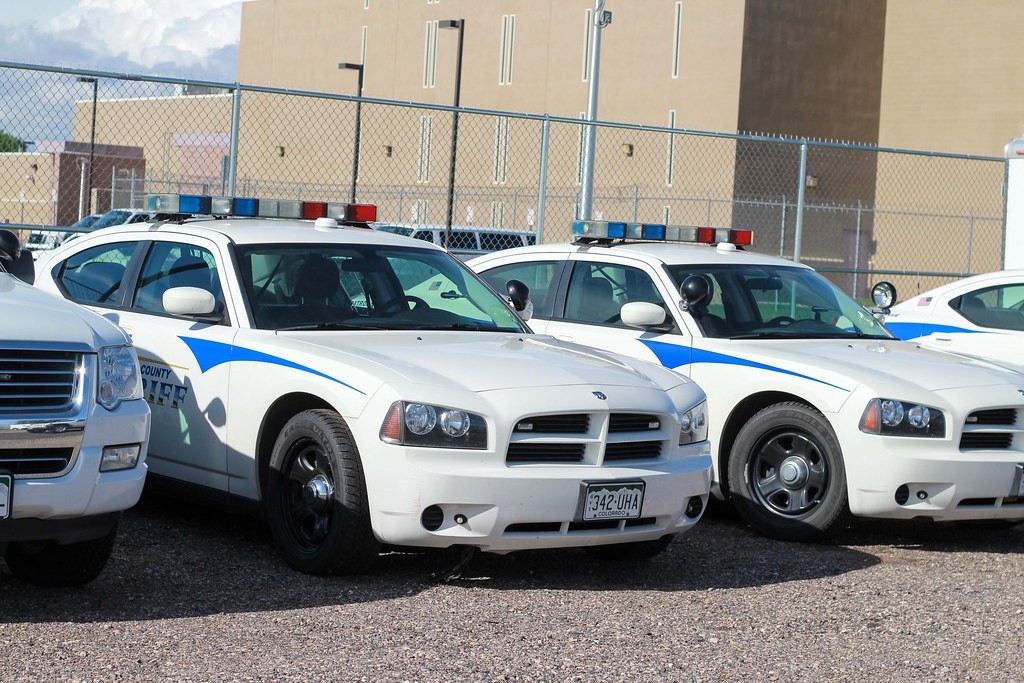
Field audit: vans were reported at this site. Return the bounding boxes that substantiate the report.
[370,222,537,265]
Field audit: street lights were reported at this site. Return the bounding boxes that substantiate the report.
[340,63,365,205]
[438,19,466,250]
[77,76,98,218]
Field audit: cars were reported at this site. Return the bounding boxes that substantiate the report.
[849,270,1024,373]
[0,228,152,593]
[35,196,713,573]
[27,208,158,259]
[349,219,1024,542]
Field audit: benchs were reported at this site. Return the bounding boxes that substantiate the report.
[80,260,279,309]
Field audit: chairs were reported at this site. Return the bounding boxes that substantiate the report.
[168,256,212,293]
[280,255,342,328]
[690,273,727,340]
[961,295,989,328]
[577,275,623,325]
